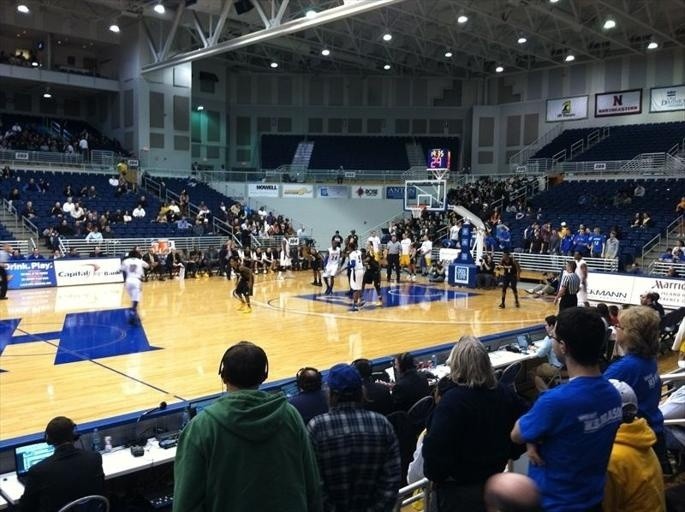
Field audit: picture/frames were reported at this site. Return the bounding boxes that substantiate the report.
[649,84,685,113]
[545,94,589,122]
[594,88,642,117]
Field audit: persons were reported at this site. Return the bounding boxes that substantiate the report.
[0,245,13,299]
[0,42,318,287]
[318,164,685,307]
[228,255,254,313]
[172,306,684,511]
[18,416,108,512]
[120,251,150,325]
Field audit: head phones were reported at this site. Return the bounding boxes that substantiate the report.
[217,343,268,384]
[45,416,79,445]
[296,367,322,391]
[398,351,410,370]
[350,359,373,375]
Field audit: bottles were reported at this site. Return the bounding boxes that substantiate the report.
[431,355,436,368]
[91,429,100,450]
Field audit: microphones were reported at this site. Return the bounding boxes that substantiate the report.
[130,401,166,456]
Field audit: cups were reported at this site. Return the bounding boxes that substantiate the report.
[104,436,112,451]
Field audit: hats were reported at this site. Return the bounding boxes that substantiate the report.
[322,363,363,389]
[608,378,638,415]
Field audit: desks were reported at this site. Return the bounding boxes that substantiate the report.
[385,339,544,386]
[0,429,177,504]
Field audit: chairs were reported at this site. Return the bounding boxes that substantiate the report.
[407,395,433,415]
[658,307,685,355]
[58,495,110,512]
[500,361,524,394]
[442,177,685,265]
[0,111,292,241]
[260,132,462,174]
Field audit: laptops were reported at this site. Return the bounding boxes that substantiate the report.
[515,332,539,350]
[15,441,56,487]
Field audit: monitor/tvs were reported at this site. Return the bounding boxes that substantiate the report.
[189,395,220,421]
